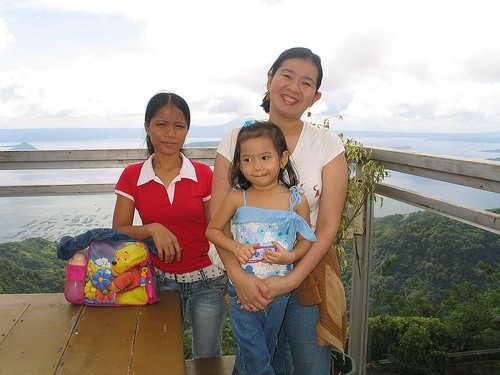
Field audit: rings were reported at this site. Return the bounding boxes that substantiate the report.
[250,305,255,310]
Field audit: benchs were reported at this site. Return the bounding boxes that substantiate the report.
[185,355,236,375]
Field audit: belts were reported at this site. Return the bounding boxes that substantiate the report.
[153,263,225,283]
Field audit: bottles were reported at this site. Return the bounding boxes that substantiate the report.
[64,252,87,304]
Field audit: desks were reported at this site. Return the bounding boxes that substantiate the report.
[0,289,187,375]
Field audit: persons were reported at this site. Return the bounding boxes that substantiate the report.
[209,47,348,375]
[113,92,230,375]
[205,121,314,375]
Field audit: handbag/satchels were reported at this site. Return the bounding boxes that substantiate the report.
[64,239,160,307]
[207,127,239,271]
